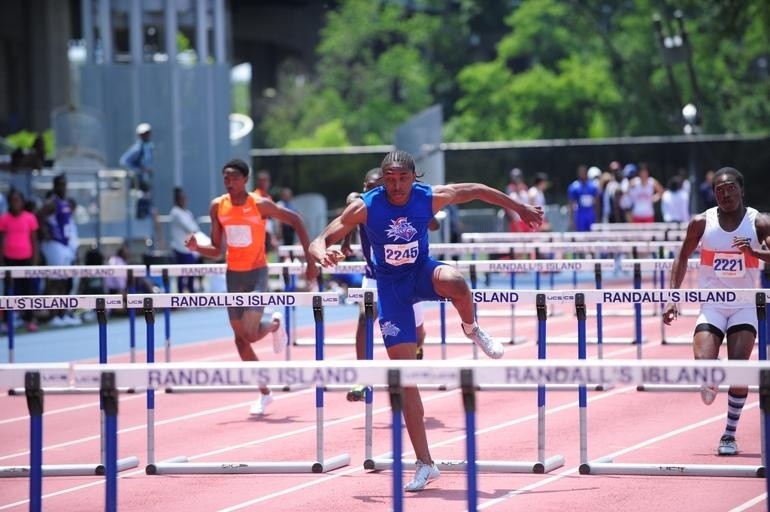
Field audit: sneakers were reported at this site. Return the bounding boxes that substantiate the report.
[347,346,422,402]
[271,312,288,353]
[0,313,80,332]
[403,459,442,492]
[250,391,274,415]
[461,323,505,360]
[702,386,718,406]
[718,436,738,457]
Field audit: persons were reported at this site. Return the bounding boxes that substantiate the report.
[275,187,298,245]
[660,167,770,456]
[183,157,320,417]
[339,168,427,401]
[501,160,717,276]
[307,150,544,493]
[252,169,278,249]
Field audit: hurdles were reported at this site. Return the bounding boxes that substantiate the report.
[1,263,152,396]
[278,242,386,347]
[74,360,436,512]
[0,293,141,476]
[436,359,770,512]
[125,290,351,475]
[1,362,71,512]
[455,257,617,393]
[621,258,765,392]
[428,221,762,347]
[342,286,570,476]
[303,260,456,390]
[149,262,312,392]
[547,288,769,478]
